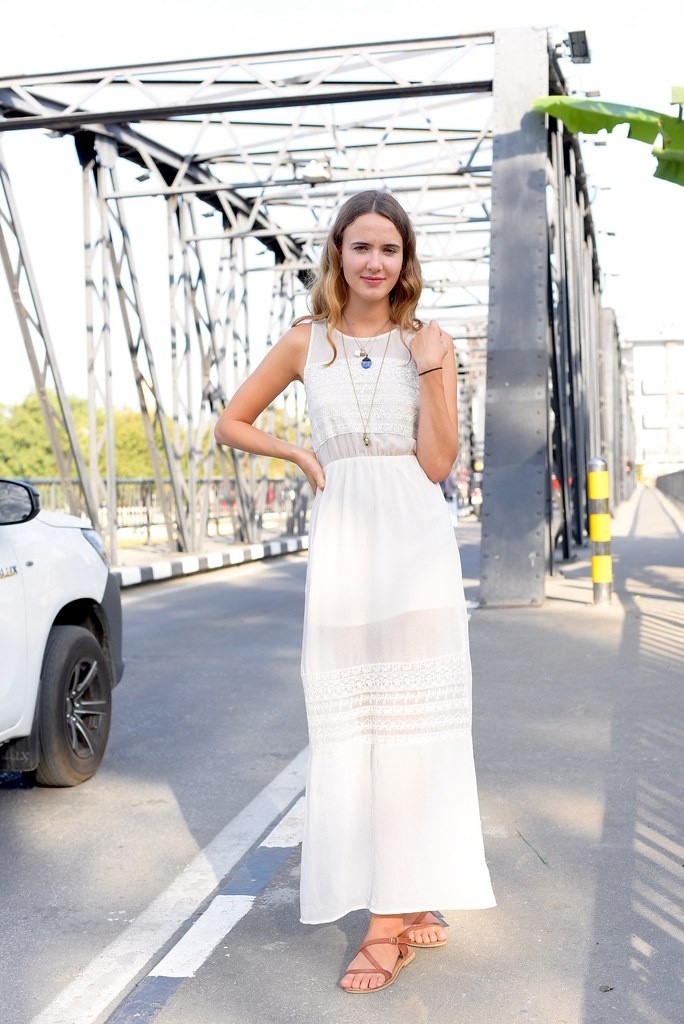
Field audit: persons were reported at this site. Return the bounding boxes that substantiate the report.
[214,190,498,992]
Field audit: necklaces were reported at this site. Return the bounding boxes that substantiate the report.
[339,309,394,448]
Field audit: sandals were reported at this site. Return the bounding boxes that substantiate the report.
[343,933,416,994]
[404,911,447,948]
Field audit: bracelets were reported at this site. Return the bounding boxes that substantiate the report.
[419,367,442,376]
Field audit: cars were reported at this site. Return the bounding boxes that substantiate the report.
[1,479,127,787]
